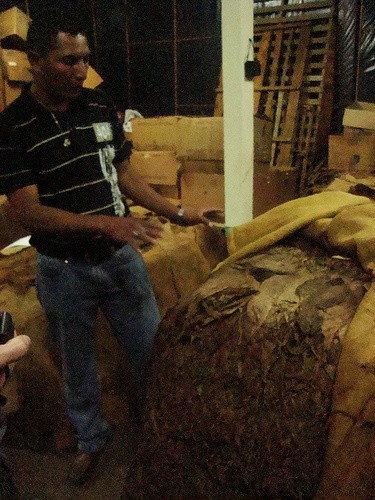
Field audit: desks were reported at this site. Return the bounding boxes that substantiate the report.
[0,211,212,450]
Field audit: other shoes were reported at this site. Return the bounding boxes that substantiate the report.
[68,450,98,485]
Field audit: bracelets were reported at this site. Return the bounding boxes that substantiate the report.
[173,208,186,225]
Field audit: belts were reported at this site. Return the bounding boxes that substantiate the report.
[35,234,120,260]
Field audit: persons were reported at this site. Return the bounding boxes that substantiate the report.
[0,8,224,485]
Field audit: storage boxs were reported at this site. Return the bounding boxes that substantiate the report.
[0,6,34,114]
[123,116,298,226]
[329,102,375,171]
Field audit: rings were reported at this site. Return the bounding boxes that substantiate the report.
[133,230,139,236]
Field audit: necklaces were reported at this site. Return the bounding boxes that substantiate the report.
[35,100,72,146]
